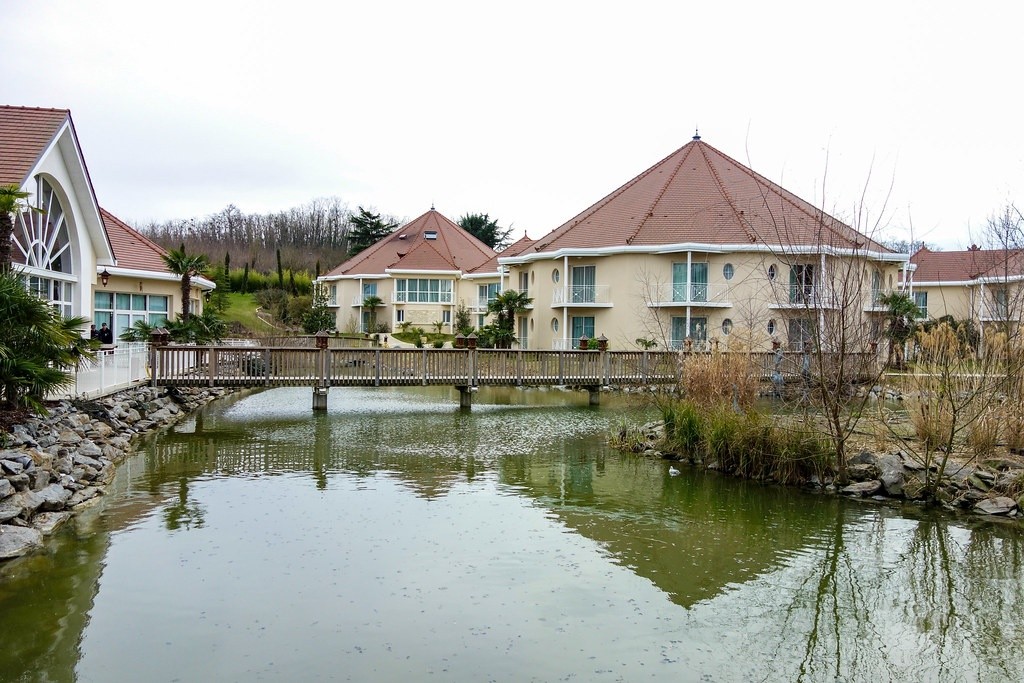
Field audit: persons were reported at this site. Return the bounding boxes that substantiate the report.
[91,324,100,356]
[98,322,112,355]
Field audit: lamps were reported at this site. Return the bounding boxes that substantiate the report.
[466,332,478,349]
[803,338,814,353]
[99,269,111,287]
[293,329,299,336]
[285,327,290,336]
[455,332,466,348]
[597,333,608,351]
[204,292,211,304]
[870,341,878,354]
[682,335,693,352]
[770,336,782,351]
[708,337,720,352]
[149,326,163,346]
[160,328,170,346]
[318,331,329,348]
[579,335,589,350]
[314,331,322,348]
